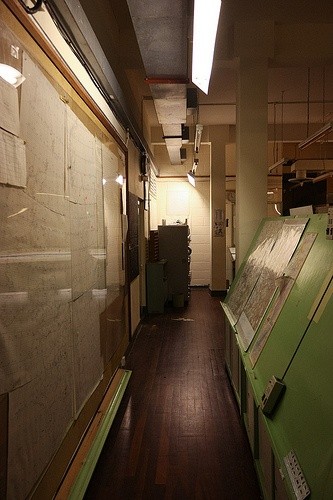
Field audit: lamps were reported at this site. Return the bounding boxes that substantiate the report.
[268,91,288,171]
[298,65,333,150]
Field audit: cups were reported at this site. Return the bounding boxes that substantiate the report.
[162,220,166,225]
